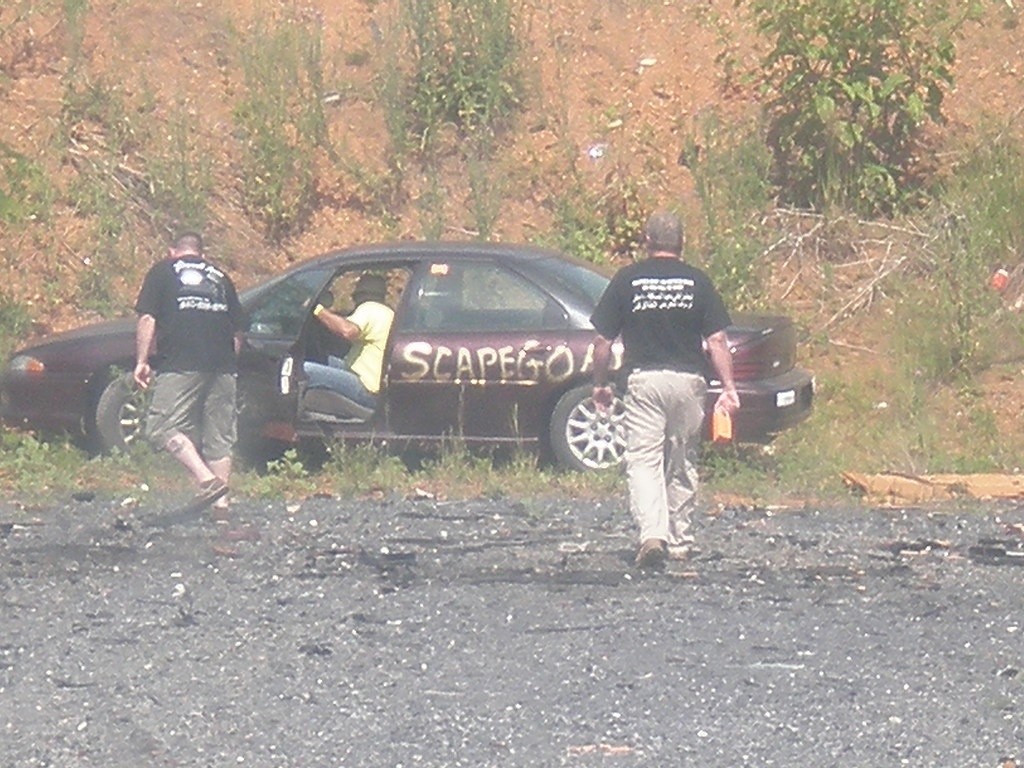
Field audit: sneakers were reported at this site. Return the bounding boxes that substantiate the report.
[635,539,691,569]
[187,479,231,525]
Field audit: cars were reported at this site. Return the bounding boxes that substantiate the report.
[1,240,817,480]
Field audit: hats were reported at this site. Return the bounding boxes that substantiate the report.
[350,273,389,296]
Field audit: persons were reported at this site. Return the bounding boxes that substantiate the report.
[589,209,742,568]
[297,271,396,412]
[133,232,252,525]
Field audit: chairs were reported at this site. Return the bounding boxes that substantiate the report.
[422,274,462,328]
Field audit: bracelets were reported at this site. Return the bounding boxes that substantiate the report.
[303,296,325,317]
